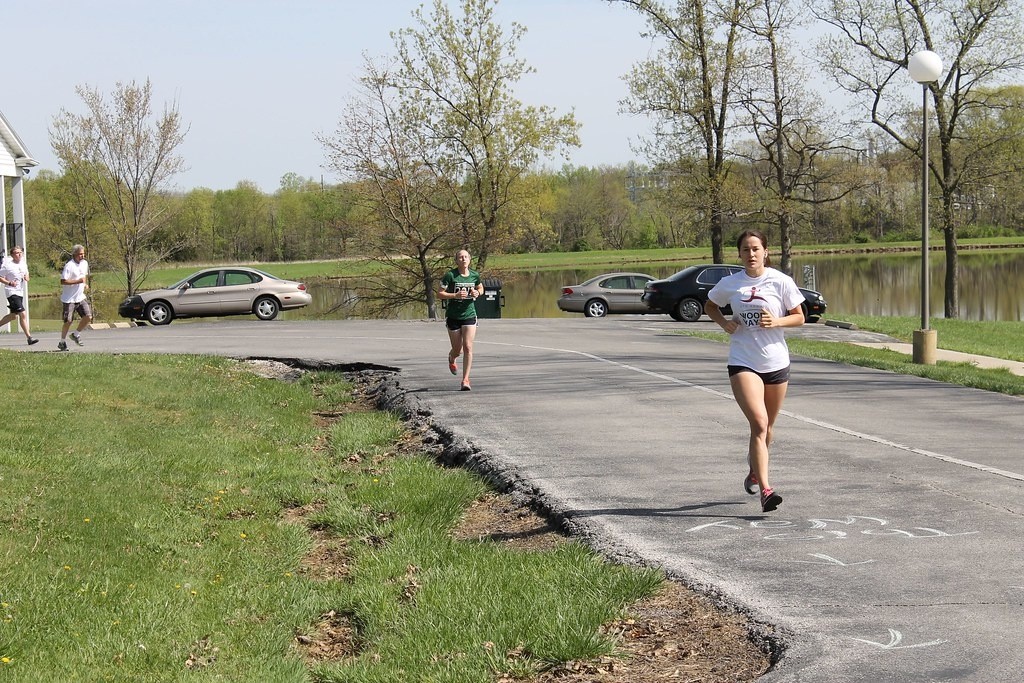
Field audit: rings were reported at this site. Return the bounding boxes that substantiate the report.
[770,322,771,324]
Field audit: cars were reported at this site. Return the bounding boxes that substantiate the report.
[556,272,665,318]
[117,267,312,325]
[641,263,827,324]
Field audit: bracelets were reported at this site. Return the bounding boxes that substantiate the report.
[478,291,480,296]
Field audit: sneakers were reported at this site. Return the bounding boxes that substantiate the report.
[58,341,69,351]
[744,453,760,495]
[69,332,84,346]
[761,488,783,512]
[449,355,457,375]
[28,336,39,345]
[460,381,471,391]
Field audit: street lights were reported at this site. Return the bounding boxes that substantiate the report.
[908,51,944,365]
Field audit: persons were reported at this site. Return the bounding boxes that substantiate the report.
[438,250,484,390]
[0,246,39,344]
[704,229,806,511]
[57,245,92,351]
